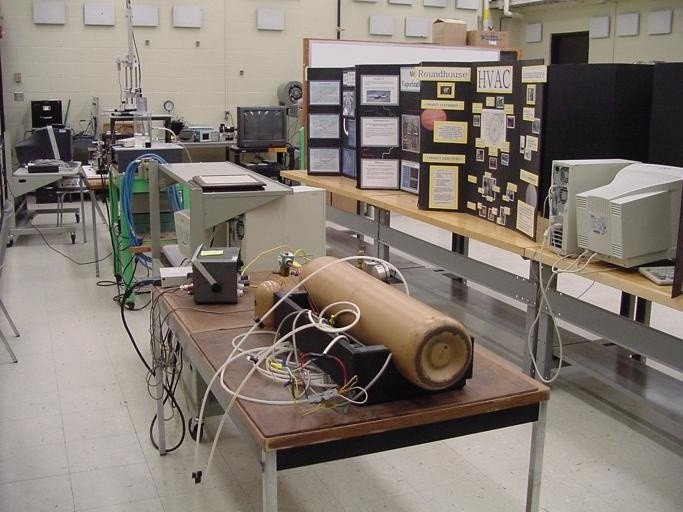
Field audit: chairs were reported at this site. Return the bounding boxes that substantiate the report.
[0,200,21,364]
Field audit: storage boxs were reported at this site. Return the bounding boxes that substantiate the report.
[469,30,507,49]
[432,17,468,46]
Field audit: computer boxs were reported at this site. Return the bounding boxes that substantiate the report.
[549,159,638,255]
[31,128,72,162]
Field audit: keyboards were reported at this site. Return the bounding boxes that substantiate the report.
[639,265,674,286]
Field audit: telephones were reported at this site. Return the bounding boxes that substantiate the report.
[200,131,220,142]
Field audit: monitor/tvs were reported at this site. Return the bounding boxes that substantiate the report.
[237,106,287,147]
[574,162,683,269]
[15,125,60,166]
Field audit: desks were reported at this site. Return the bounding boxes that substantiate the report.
[80,165,119,277]
[365,193,557,378]
[280,169,417,276]
[526,239,683,458]
[7,161,83,244]
[148,161,294,279]
[151,283,551,511]
